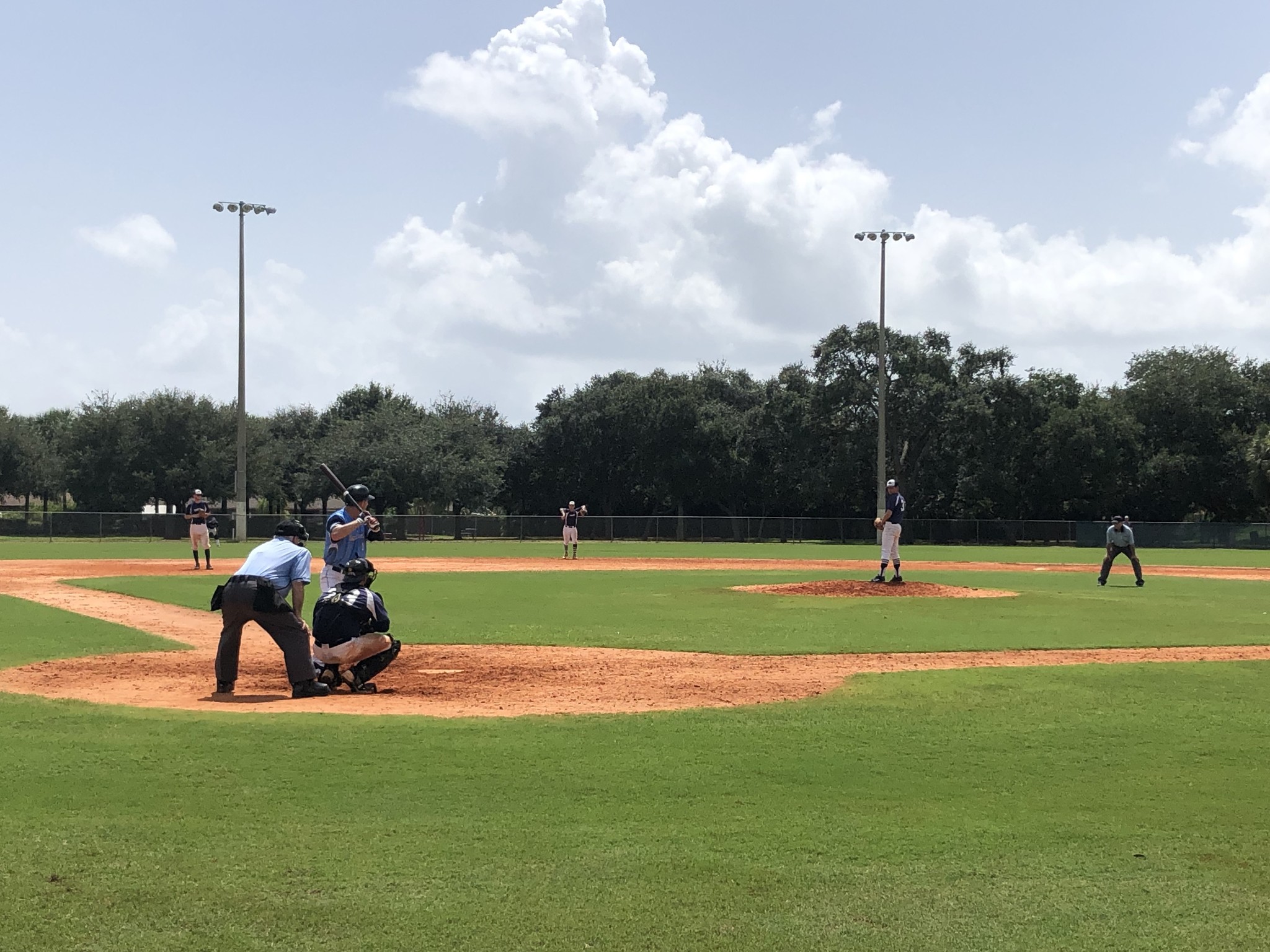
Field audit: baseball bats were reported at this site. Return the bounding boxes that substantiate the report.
[319,463,380,531]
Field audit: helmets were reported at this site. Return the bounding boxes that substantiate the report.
[343,484,375,506]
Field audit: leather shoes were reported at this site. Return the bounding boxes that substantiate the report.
[217,681,235,693]
[292,682,330,699]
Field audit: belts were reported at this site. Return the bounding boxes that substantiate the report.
[325,564,345,574]
[196,523,205,525]
[314,637,352,648]
[566,526,575,528]
[230,575,259,582]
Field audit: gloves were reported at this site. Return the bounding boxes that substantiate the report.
[357,510,380,533]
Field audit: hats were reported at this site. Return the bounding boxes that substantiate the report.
[1124,516,1129,519]
[275,519,306,536]
[193,489,202,495]
[569,501,575,505]
[342,558,375,583]
[885,479,898,489]
[1111,516,1123,523]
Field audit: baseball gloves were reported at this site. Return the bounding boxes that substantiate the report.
[874,517,885,531]
[199,508,212,519]
[580,508,587,518]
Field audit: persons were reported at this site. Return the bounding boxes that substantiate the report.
[870,479,906,585]
[184,488,213,570]
[315,484,385,678]
[215,520,329,699]
[560,501,586,559]
[1096,515,1145,587]
[207,517,221,547]
[1122,516,1134,533]
[312,558,401,693]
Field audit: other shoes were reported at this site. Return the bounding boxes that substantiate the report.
[572,554,577,560]
[563,554,568,559]
[889,575,903,582]
[194,563,200,570]
[1098,577,1107,586]
[1135,579,1145,587]
[206,564,213,570]
[871,573,885,582]
[320,667,372,694]
